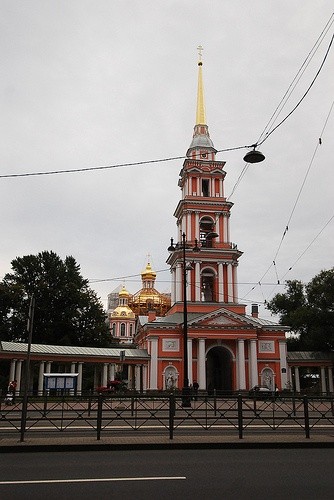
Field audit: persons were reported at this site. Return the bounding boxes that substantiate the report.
[190,384,193,401]
[5,381,15,406]
[166,371,174,389]
[264,371,271,388]
[193,380,199,401]
[275,386,282,402]
[12,380,17,405]
